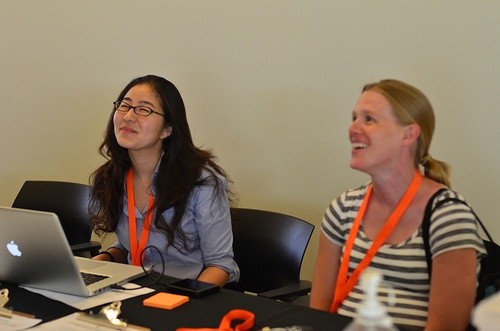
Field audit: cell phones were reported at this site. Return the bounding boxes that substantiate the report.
[165,277,220,298]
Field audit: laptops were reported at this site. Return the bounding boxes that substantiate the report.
[0,207,152,297]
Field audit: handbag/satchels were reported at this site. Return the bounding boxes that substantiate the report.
[423,187,500,306]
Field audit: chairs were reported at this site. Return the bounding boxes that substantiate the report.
[222,208,315,299]
[12,179,104,257]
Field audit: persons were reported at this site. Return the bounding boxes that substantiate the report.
[308,79,488,331]
[88,75,240,288]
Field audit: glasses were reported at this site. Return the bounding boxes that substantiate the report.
[113,100,166,118]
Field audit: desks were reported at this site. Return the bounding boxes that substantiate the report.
[0,271,353,331]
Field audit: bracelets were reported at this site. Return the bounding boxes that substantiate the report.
[98,250,115,262]
